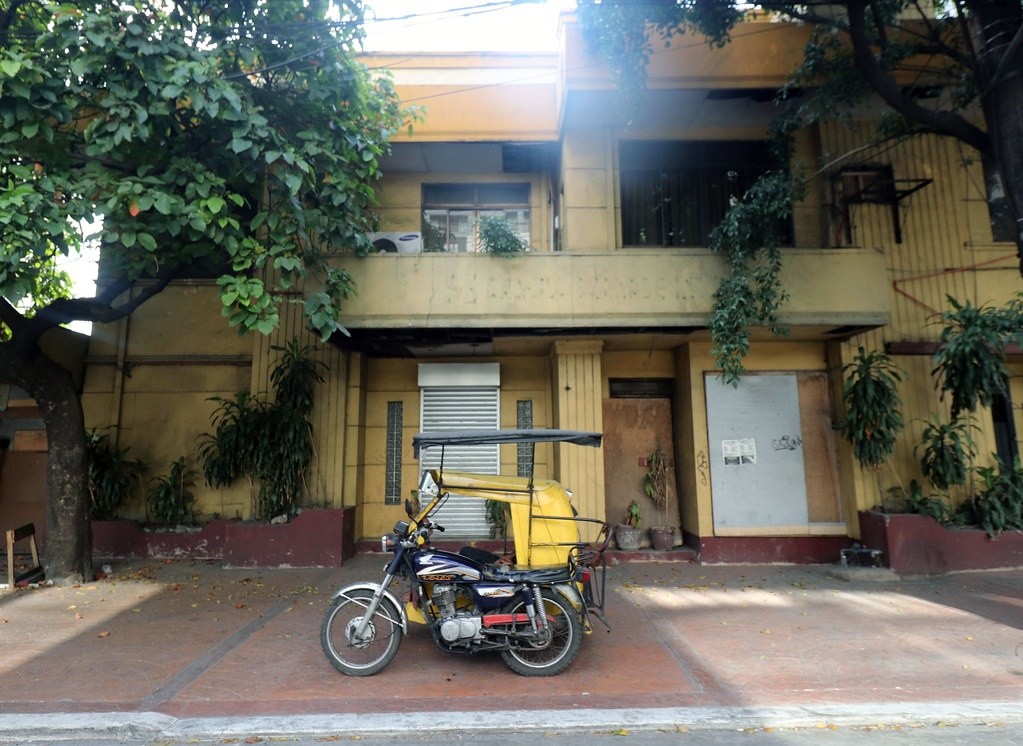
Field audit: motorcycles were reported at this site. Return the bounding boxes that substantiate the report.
[319,429,615,677]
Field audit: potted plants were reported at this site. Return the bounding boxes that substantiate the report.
[614,500,643,552]
[642,449,676,550]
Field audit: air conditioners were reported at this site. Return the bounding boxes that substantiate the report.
[355,230,425,253]
